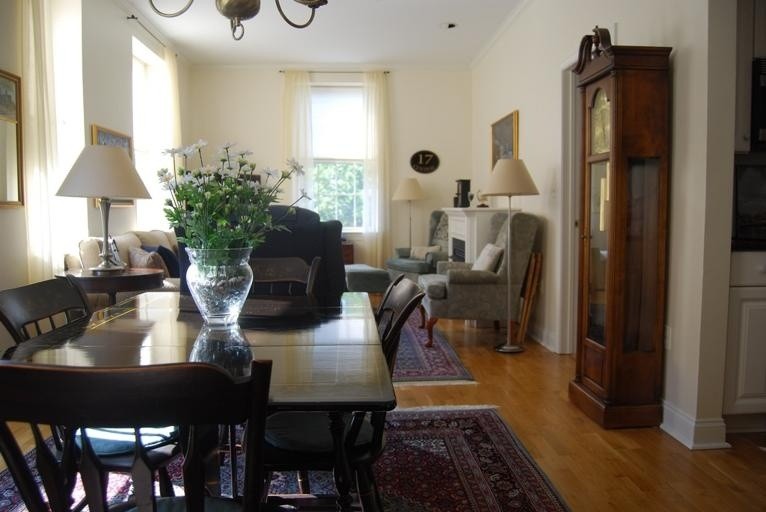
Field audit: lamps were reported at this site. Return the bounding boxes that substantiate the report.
[53,144,155,272]
[480,157,545,356]
[392,175,427,248]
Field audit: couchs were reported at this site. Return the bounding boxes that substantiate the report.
[62,230,184,313]
[173,194,350,295]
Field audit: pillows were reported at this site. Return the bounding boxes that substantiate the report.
[408,245,441,260]
[125,244,177,278]
[471,241,503,274]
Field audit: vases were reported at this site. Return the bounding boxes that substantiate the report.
[181,245,259,326]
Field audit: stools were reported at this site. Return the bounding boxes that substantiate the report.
[343,264,392,295]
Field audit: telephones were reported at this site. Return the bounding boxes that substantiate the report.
[109,234,128,267]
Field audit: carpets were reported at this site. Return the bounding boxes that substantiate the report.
[369,294,480,386]
[0,401,577,512]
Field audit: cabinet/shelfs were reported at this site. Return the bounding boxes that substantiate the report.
[567,42,670,433]
[442,205,522,264]
[725,240,766,437]
[342,238,353,265]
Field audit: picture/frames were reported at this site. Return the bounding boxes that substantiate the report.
[89,122,140,211]
[489,108,520,167]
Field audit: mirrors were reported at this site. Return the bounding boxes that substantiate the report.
[0,66,26,211]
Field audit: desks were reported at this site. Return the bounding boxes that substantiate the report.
[54,267,167,312]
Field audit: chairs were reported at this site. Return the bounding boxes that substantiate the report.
[0,270,194,498]
[416,206,540,349]
[0,355,276,512]
[384,207,449,284]
[233,255,321,297]
[243,268,424,508]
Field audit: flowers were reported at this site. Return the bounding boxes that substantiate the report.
[154,140,313,282]
